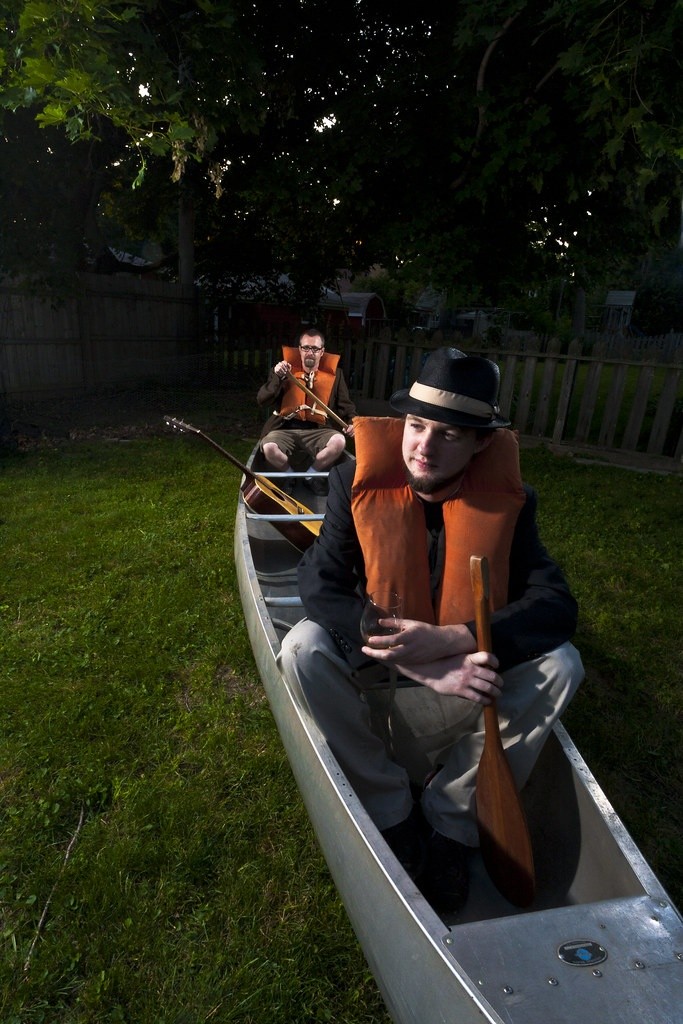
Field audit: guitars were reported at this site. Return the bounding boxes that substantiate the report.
[163,414,324,554]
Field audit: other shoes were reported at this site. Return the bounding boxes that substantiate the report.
[279,471,298,496]
[302,476,329,496]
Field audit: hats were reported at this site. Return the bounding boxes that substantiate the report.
[389,346,512,427]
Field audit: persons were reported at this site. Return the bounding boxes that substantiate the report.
[272,343,586,925]
[254,328,359,498]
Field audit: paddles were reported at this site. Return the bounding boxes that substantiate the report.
[470,555,539,910]
[284,367,349,429]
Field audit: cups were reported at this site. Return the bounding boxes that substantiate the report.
[359,592,404,650]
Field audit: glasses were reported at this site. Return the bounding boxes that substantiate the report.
[298,343,324,352]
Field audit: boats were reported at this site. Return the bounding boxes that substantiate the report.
[235,412,683,1023]
